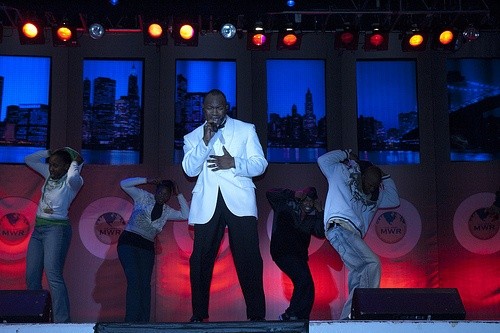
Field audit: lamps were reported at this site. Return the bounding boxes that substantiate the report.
[89,21,107,40]
[247,22,272,52]
[221,23,237,40]
[399,24,428,52]
[52,21,79,47]
[277,21,303,51]
[174,22,199,47]
[335,29,360,51]
[431,26,461,54]
[363,26,389,51]
[462,24,480,41]
[143,21,169,46]
[18,19,45,45]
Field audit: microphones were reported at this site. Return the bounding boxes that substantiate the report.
[210,117,218,132]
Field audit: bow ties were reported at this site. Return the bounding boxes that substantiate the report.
[217,117,228,129]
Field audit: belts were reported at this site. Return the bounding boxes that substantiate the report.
[327,221,340,229]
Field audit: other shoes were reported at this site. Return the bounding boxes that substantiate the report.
[278,311,290,321]
[191,314,203,322]
[248,315,266,320]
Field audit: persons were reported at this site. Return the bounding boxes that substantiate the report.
[181,89,269,323]
[116,173,189,323]
[267,184,326,319]
[317,146,401,320]
[25,146,84,323]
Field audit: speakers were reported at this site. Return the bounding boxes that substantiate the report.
[351,286,466,320]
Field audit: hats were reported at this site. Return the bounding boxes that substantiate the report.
[364,166,382,185]
